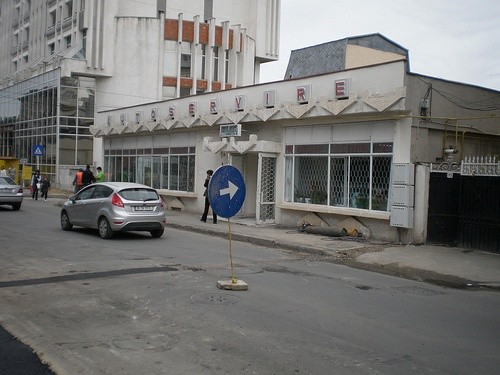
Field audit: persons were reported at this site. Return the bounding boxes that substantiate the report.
[200,170,217,224]
[73,164,104,193]
[31,169,50,202]
[0,164,16,182]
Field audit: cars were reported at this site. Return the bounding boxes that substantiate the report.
[0,176,23,210]
[60,181,165,238]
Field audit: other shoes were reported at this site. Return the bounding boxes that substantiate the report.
[44,199,47,201]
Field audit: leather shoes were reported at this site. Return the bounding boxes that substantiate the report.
[213,221,217,224]
[199,219,206,222]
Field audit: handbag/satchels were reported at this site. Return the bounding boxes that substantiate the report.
[36,180,43,189]
